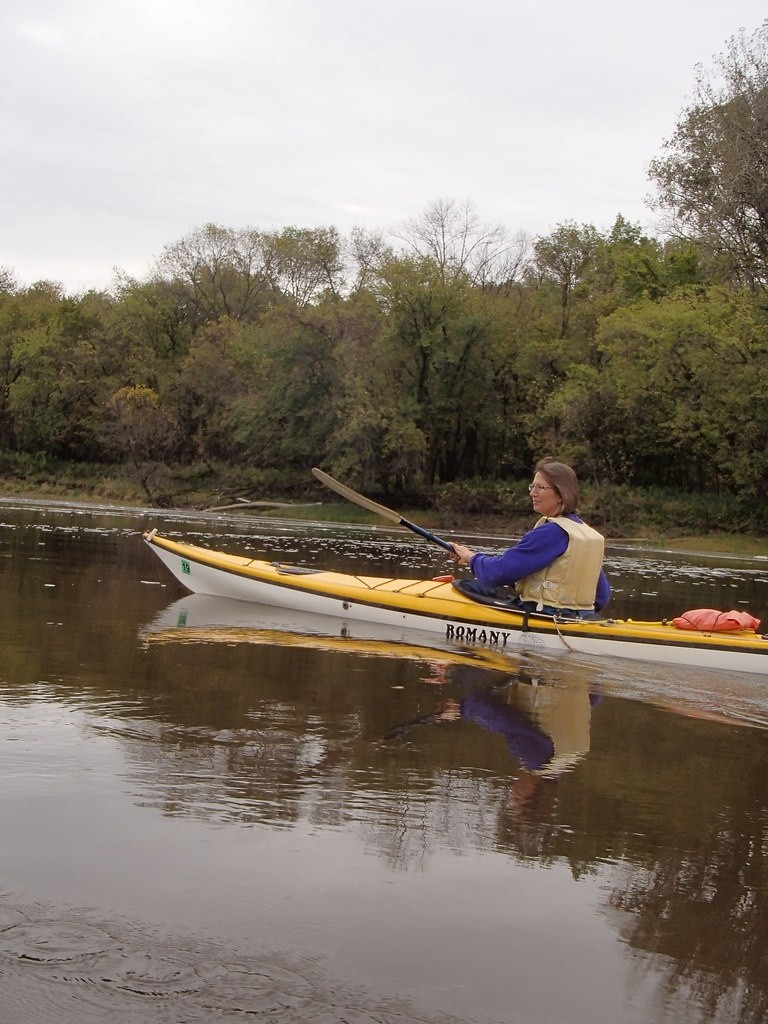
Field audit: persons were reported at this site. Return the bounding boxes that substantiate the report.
[447,462,610,620]
[435,674,600,830]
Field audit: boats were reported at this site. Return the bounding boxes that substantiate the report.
[140,528,768,675]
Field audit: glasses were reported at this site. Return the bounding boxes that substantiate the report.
[528,483,552,491]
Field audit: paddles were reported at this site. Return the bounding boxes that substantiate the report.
[310,466,456,554]
